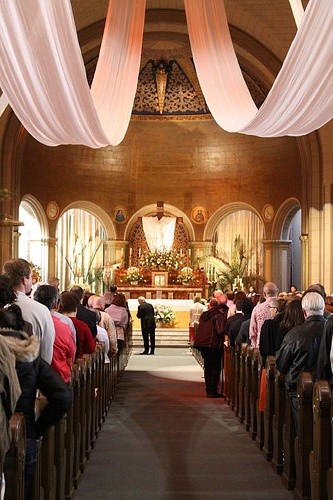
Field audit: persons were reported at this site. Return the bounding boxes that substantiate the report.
[195,304,230,397]
[0,258,132,500]
[137,296,156,354]
[189,282,333,433]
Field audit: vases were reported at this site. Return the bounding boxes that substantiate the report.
[151,265,169,272]
[183,282,189,286]
[130,281,138,285]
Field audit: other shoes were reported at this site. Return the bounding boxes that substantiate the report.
[138,351,153,355]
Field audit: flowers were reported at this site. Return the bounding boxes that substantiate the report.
[120,267,148,283]
[177,267,199,286]
[153,303,176,324]
[139,247,187,272]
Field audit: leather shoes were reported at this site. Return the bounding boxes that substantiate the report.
[206,391,223,398]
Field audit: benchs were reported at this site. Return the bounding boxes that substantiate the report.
[191,321,333,500]
[0,341,119,500]
